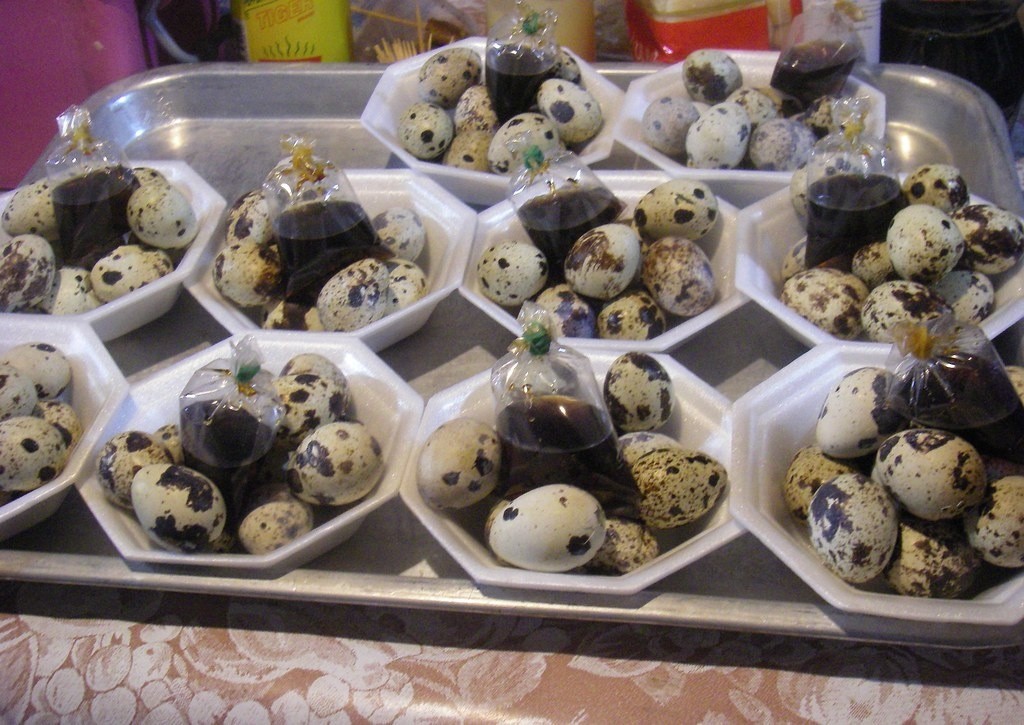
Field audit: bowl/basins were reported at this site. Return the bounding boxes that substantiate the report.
[0,35,1024,649]
